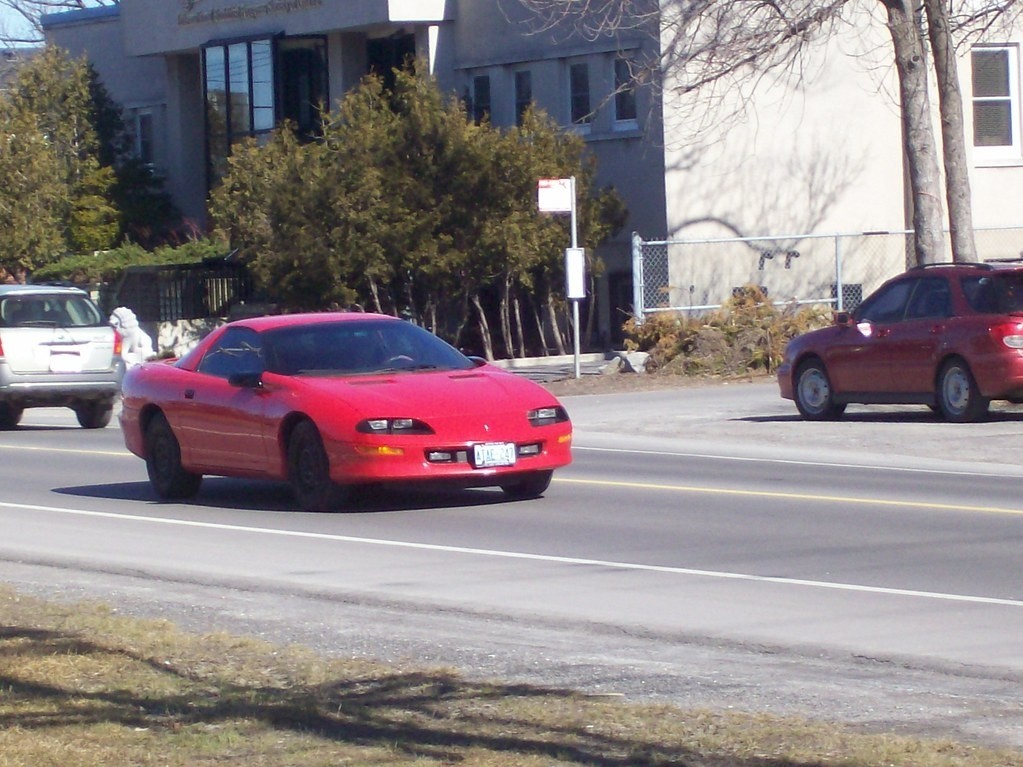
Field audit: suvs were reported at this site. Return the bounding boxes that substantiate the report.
[0,282,127,430]
[778,258,1023,424]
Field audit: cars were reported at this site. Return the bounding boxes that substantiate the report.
[117,312,572,513]
